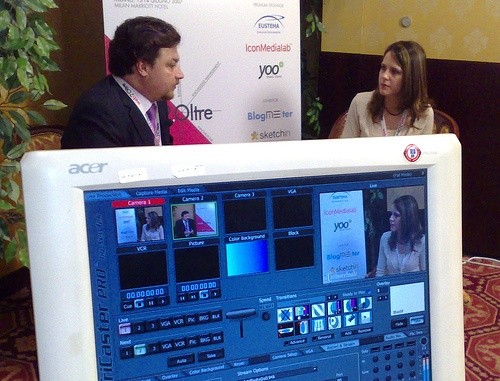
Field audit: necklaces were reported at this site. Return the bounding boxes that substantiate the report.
[395,246,410,274]
[379,109,409,137]
[384,106,404,116]
[397,241,408,244]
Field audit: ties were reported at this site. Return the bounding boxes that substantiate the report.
[146,104,160,146]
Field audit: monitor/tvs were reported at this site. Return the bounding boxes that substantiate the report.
[22,132,465,381]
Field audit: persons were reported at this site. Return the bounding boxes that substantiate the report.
[341,40,435,138]
[60,15,185,150]
[139,211,164,241]
[375,195,426,277]
[174,210,197,239]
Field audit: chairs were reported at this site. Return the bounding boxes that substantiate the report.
[328,107,459,140]
[0,126,66,284]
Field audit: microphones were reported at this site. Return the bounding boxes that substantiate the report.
[165,119,173,126]
